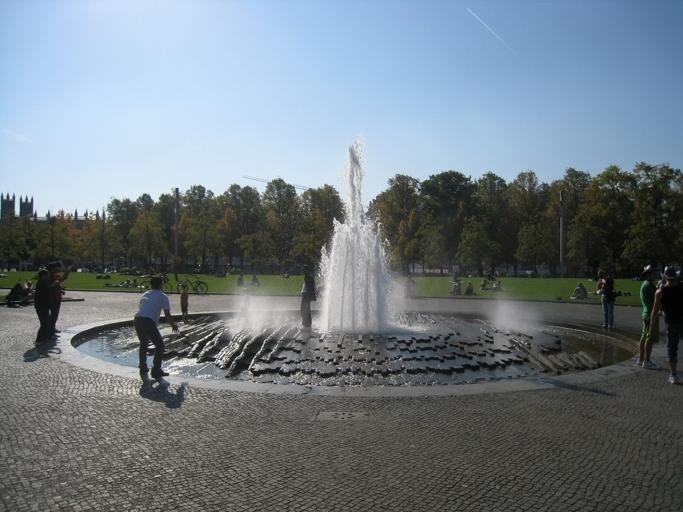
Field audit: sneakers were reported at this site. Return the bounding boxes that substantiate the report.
[601,324,612,329]
[637,357,680,384]
[36,328,61,343]
[141,368,169,376]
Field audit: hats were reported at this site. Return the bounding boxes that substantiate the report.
[665,266,676,279]
[641,264,661,277]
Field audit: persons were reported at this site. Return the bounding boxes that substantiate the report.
[132,276,180,376]
[570,263,683,386]
[19,280,34,295]
[103,279,130,289]
[237,273,243,287]
[43,260,63,336]
[33,270,60,343]
[449,265,501,297]
[179,282,188,316]
[250,272,257,284]
[404,275,415,295]
[114,279,139,288]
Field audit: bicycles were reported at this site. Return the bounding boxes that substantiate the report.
[140,274,172,294]
[177,274,208,296]
